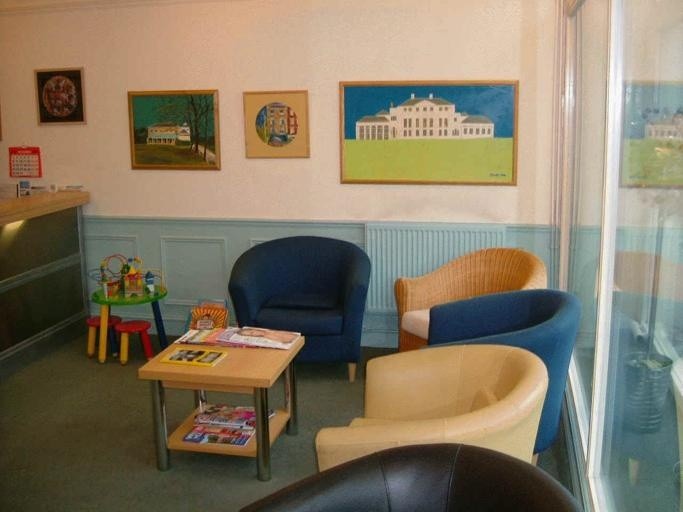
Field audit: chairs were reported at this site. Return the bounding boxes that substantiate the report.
[237,441,587,511]
[311,341,551,472]
[226,232,371,384]
[392,243,550,354]
[425,286,583,467]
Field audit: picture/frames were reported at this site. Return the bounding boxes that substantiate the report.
[32,65,88,128]
[126,89,221,172]
[617,79,682,193]
[336,78,521,188]
[241,89,311,160]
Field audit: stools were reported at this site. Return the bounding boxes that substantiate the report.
[85,314,122,356]
[113,319,153,367]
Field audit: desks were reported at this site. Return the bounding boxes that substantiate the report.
[137,332,307,482]
[90,284,168,364]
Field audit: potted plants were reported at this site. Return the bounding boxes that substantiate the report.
[615,102,681,438]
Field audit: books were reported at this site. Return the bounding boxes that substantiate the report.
[183,402,279,446]
[160,346,228,367]
[177,326,302,349]
